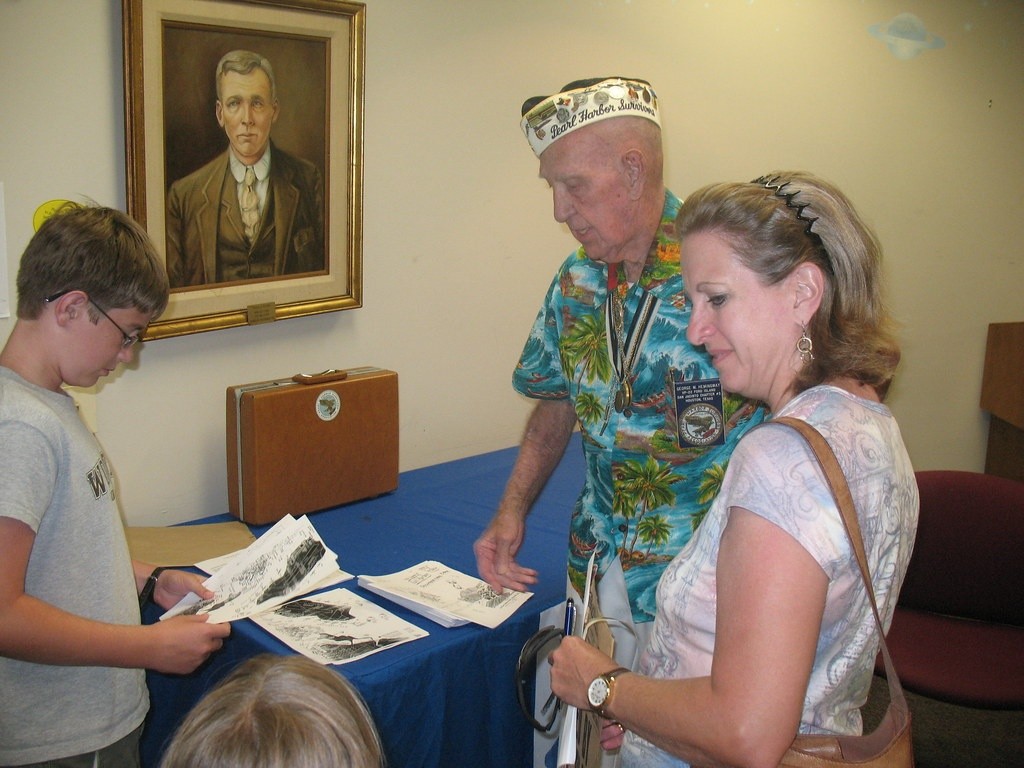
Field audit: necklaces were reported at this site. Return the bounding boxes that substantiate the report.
[612,285,652,413]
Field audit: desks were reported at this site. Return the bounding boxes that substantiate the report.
[125,429,585,768]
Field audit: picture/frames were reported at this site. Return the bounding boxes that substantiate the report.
[120,0,365,339]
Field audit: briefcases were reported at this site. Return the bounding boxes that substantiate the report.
[224,366,398,527]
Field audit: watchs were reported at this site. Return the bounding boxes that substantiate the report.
[589,667,629,719]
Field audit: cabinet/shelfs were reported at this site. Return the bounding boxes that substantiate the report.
[979,321,1024,484]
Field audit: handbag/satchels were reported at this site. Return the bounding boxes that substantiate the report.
[780,685,916,768]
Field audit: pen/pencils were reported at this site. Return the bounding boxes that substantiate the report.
[562,598,575,636]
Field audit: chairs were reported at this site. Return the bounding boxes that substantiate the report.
[871,469,1024,715]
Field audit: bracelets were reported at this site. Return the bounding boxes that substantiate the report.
[139,567,163,605]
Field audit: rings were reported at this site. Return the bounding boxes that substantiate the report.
[617,723,625,732]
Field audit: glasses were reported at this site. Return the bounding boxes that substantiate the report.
[51,289,145,348]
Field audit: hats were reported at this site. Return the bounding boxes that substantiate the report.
[523,76,664,156]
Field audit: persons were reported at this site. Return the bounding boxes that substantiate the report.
[161,655,385,767]
[547,169,918,768]
[0,206,229,768]
[474,75,772,674]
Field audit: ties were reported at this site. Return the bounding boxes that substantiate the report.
[240,167,261,244]
